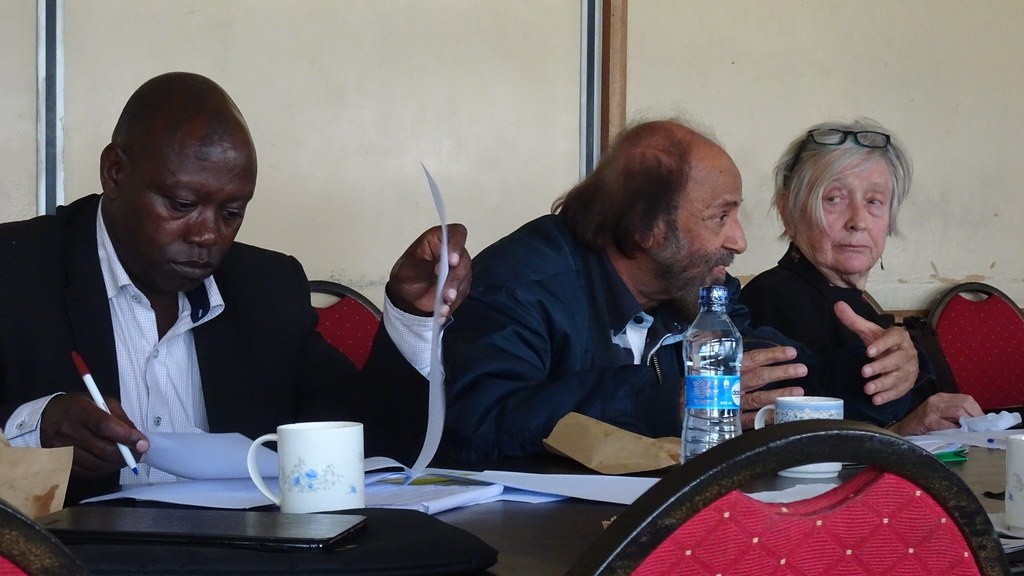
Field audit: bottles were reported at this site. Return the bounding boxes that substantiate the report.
[679,286,743,467]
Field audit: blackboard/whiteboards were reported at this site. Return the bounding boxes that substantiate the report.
[602,0,1024,324]
[35,0,610,315]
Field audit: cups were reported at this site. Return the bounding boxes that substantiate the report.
[1005,434,1024,533]
[247,421,365,514]
[754,396,845,479]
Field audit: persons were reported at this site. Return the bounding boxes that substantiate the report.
[0,71,472,508]
[742,117,986,437]
[435,121,920,465]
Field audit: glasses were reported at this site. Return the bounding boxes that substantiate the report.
[783,129,890,190]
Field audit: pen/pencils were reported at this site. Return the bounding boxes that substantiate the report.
[986,438,1006,445]
[71,348,140,478]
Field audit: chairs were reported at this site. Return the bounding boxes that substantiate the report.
[307,279,384,372]
[564,419,1015,576]
[928,282,1024,428]
